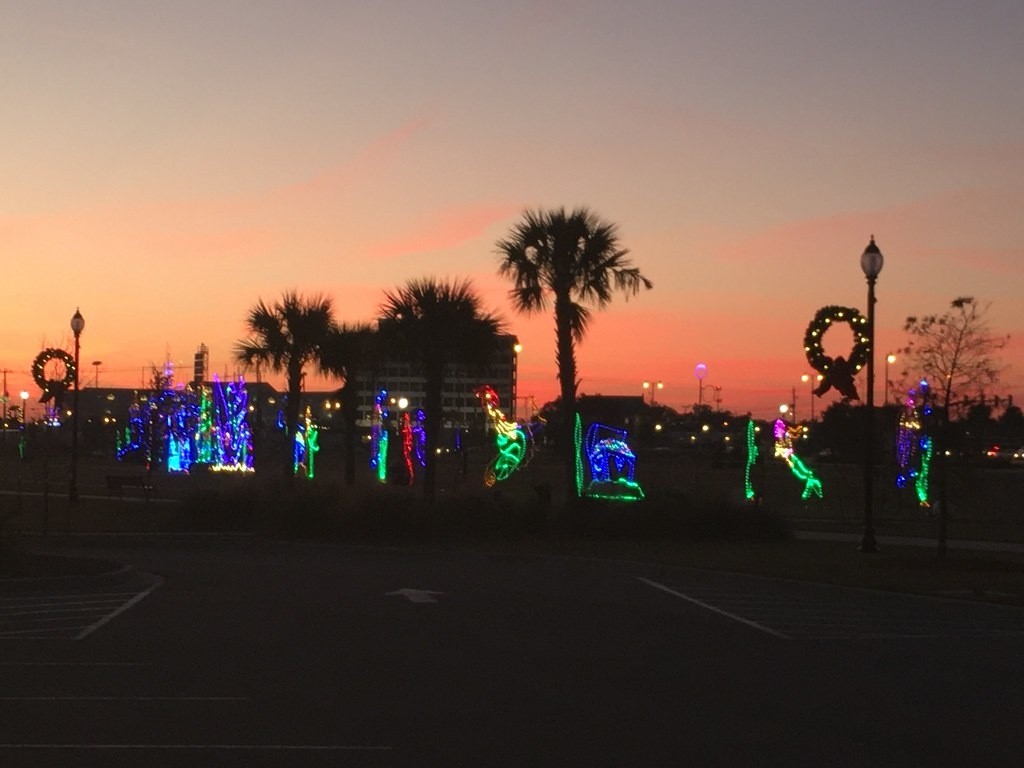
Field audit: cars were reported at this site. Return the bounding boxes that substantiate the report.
[980,445,1024,469]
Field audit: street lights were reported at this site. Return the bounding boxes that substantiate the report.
[858,234,885,554]
[800,372,824,457]
[884,355,896,448]
[61,309,86,507]
[92,361,101,390]
[642,380,663,403]
[694,361,706,404]
